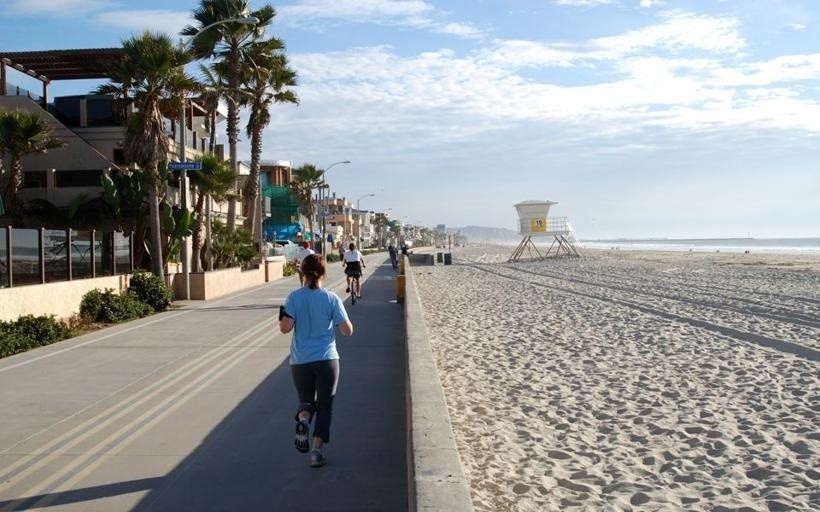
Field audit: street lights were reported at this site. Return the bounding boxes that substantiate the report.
[322,160,352,256]
[357,193,375,251]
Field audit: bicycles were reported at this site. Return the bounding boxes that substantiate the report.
[342,264,366,305]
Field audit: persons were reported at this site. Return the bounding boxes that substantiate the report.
[342,243,366,298]
[297,240,315,288]
[402,244,410,256]
[339,243,346,261]
[388,242,399,270]
[281,253,355,467]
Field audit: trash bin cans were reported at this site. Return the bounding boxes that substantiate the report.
[400,260,404,275]
[437,253,443,263]
[444,253,452,265]
[395,275,405,304]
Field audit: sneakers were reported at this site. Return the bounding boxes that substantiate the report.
[346,288,351,293]
[357,296,362,300]
[294,419,310,453]
[309,451,328,467]
